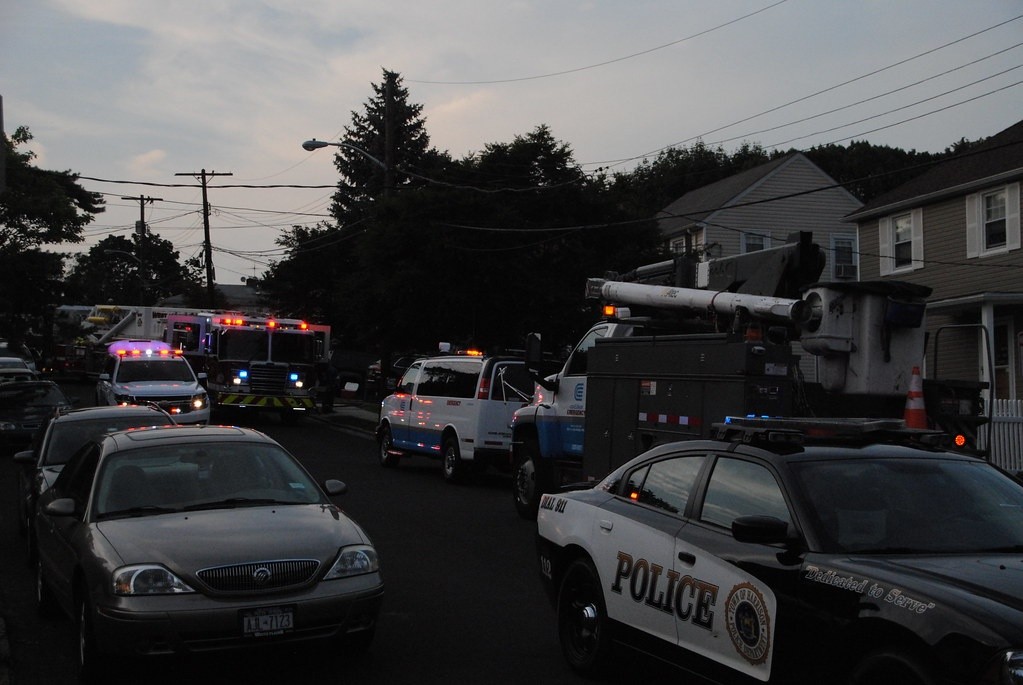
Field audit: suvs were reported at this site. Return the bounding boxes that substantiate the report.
[13,398,177,569]
[97,340,211,429]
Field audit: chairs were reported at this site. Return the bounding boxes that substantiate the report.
[107,464,153,512]
[198,449,252,498]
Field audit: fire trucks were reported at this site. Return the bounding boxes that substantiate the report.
[53,304,340,426]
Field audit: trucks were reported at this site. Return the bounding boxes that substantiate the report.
[510,229,997,523]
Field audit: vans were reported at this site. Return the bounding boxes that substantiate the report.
[374,340,564,486]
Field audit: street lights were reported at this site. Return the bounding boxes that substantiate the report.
[102,250,146,307]
[302,137,398,375]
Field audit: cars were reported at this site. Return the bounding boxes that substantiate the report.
[536,417,1023,684]
[339,351,426,404]
[35,425,387,679]
[0,337,81,453]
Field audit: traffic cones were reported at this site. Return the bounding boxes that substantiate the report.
[899,362,927,431]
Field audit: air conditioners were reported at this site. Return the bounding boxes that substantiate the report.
[835,263,857,278]
